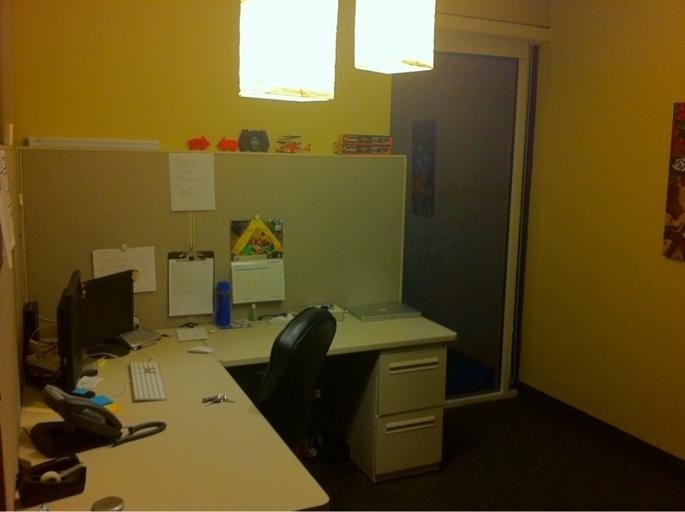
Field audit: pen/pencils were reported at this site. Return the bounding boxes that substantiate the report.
[134,342,156,351]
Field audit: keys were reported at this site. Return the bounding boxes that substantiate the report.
[201,390,236,408]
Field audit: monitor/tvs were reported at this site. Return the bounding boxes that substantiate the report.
[81,271,135,336]
[56,269,85,392]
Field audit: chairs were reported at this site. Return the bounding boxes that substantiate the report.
[227,307,336,466]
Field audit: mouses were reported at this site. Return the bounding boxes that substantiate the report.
[188,346,213,354]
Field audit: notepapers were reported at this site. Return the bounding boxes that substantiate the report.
[90,395,113,406]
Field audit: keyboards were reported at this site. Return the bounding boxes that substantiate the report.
[129,360,166,402]
[120,327,162,348]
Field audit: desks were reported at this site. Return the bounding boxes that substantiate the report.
[200,310,459,483]
[14,326,330,511]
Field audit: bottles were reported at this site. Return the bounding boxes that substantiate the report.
[214,279,233,331]
[246,303,259,324]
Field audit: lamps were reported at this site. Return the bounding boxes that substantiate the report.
[239,0,339,103]
[353,0,436,74]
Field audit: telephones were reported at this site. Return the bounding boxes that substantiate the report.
[42,384,122,439]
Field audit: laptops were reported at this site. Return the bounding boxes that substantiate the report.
[347,303,420,322]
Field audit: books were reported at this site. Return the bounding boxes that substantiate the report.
[337,132,392,146]
[175,326,210,343]
[334,143,395,156]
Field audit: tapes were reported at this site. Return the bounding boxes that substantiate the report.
[40,463,87,483]
